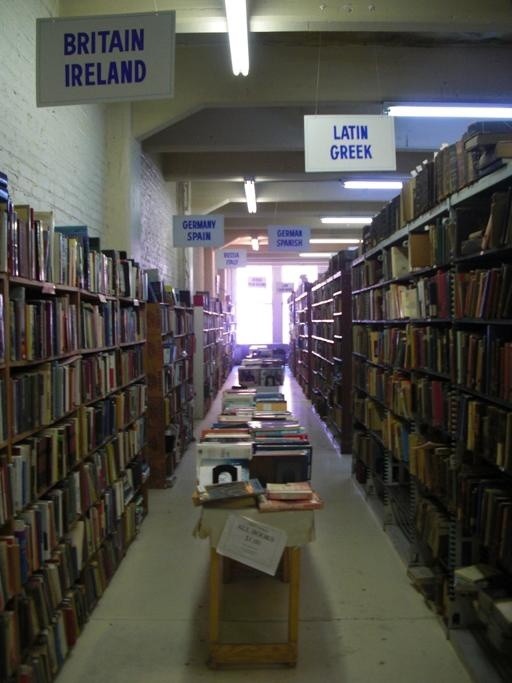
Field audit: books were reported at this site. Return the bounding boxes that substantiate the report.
[290,121,512,657]
[2,204,234,683]
[195,347,323,512]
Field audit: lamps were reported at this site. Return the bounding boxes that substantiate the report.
[248,239,262,255]
[342,176,405,191]
[307,239,362,246]
[223,0,251,76]
[384,102,511,119]
[318,217,374,224]
[298,252,336,257]
[245,182,257,214]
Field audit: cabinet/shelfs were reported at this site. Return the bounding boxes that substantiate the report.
[0,273,147,682]
[222,313,235,381]
[291,282,308,396]
[284,293,296,378]
[196,504,306,669]
[328,165,512,682]
[310,270,352,453]
[196,306,222,418]
[149,303,196,493]
